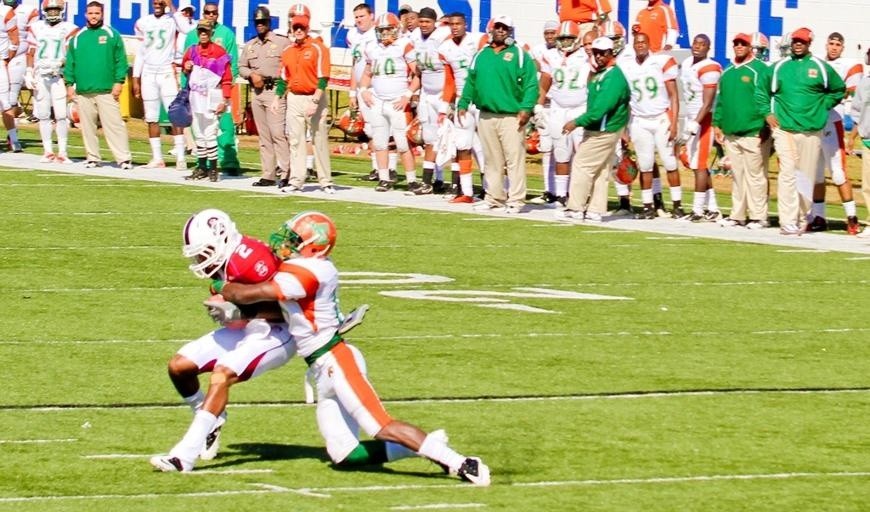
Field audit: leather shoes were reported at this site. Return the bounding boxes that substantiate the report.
[24,68,37,90]
[203,300,241,326]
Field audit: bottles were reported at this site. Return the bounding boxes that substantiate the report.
[495,15,512,29]
[591,37,615,50]
[792,29,812,43]
[177,2,311,33]
[733,34,752,45]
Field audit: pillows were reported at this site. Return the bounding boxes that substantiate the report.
[554,20,580,40]
[336,110,364,137]
[184,209,240,246]
[286,211,337,258]
[600,21,625,39]
[750,32,769,49]
[781,34,793,47]
[407,116,424,146]
[375,12,399,30]
[612,142,735,185]
[524,127,541,154]
[42,0,64,11]
[66,100,81,124]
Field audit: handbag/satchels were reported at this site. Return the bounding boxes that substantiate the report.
[211,279,231,293]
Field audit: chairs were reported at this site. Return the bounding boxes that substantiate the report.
[40,152,73,164]
[150,454,193,472]
[84,161,101,168]
[140,142,334,196]
[457,456,491,486]
[200,408,227,460]
[357,169,522,214]
[8,103,39,125]
[120,161,132,170]
[427,429,454,474]
[6,135,21,152]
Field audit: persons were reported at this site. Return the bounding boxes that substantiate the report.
[0,0,870,243]
[205,210,494,487]
[148,209,295,475]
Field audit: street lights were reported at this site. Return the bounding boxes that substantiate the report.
[168,88,192,128]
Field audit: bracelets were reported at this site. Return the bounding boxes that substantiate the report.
[211,279,231,293]
